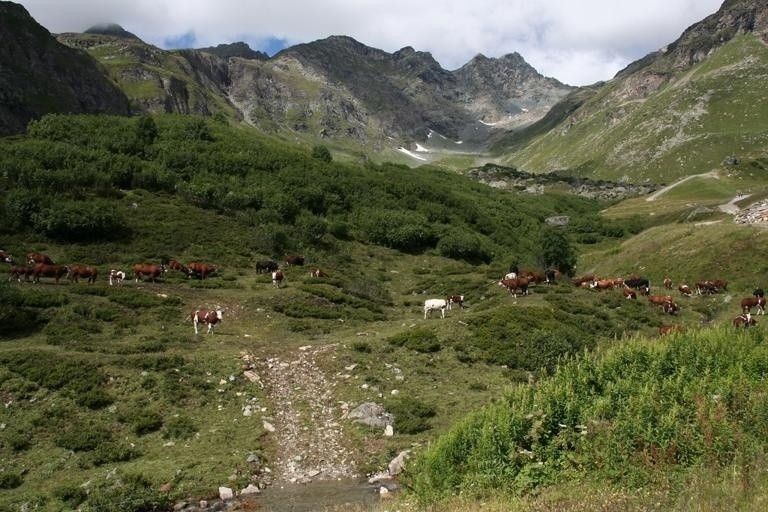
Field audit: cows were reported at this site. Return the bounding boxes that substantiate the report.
[500,263,562,298]
[733,288,767,329]
[659,326,671,337]
[255,254,329,288]
[446,295,465,312]
[0,250,98,283]
[571,273,731,316]
[423,297,446,320]
[105,257,218,286]
[190,309,225,335]
